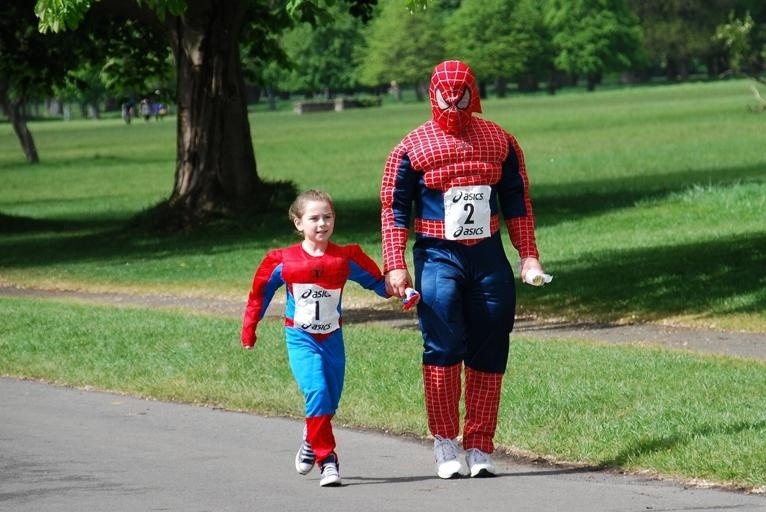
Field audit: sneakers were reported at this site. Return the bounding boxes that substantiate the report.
[294,423,317,477]
[319,454,343,488]
[432,433,499,479]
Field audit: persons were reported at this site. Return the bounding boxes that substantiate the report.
[379,58,548,480]
[120,96,168,126]
[240,189,423,488]
